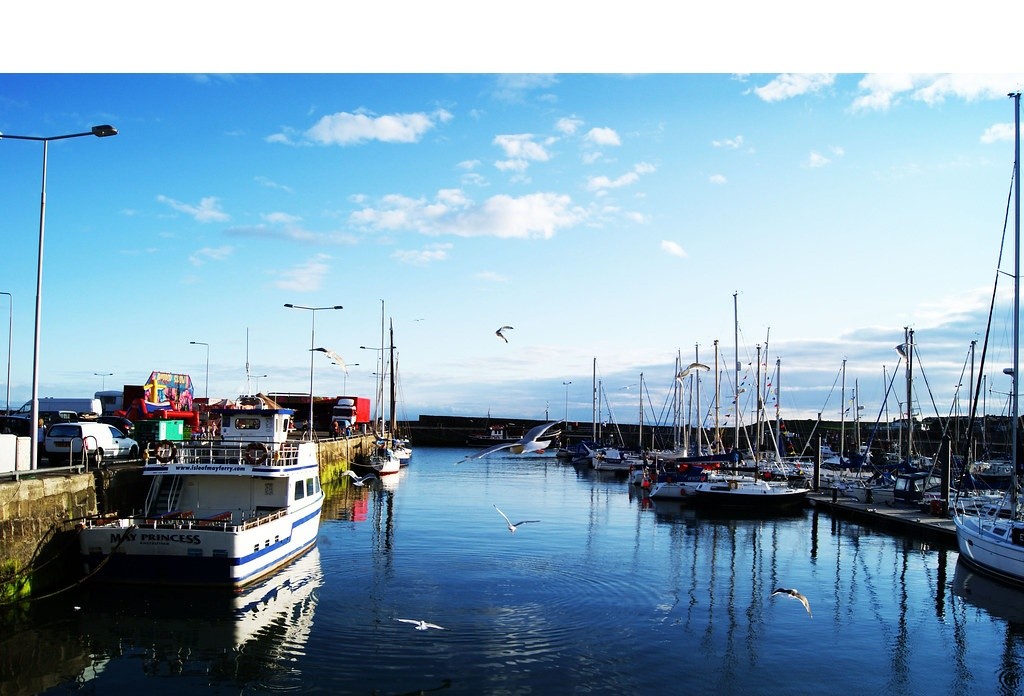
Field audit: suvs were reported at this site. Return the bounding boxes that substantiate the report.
[45,421,139,468]
[93,415,136,438]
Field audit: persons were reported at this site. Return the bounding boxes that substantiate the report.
[37,418,47,469]
[332,419,351,437]
[211,421,218,437]
[303,419,308,433]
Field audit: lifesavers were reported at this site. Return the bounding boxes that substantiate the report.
[245,442,268,465]
[154,440,176,463]
[728,481,738,489]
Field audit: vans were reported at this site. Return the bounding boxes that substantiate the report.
[0,409,79,435]
[11,396,103,418]
[94,390,125,416]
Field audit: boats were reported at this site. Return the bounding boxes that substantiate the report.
[75,543,321,672]
[77,407,326,588]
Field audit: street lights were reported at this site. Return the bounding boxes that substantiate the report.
[284,303,344,440]
[250,375,268,394]
[562,381,572,431]
[330,363,359,396]
[0,124,120,471]
[189,342,210,398]
[94,373,113,391]
[359,346,397,432]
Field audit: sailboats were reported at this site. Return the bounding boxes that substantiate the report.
[555,87,1024,588]
[362,298,412,475]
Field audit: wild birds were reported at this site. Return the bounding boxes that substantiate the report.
[676,363,710,381]
[772,587,813,619]
[396,618,450,631]
[454,418,566,465]
[493,503,540,532]
[891,342,917,364]
[496,325,514,344]
[309,347,347,373]
[619,383,637,390]
[413,319,424,322]
